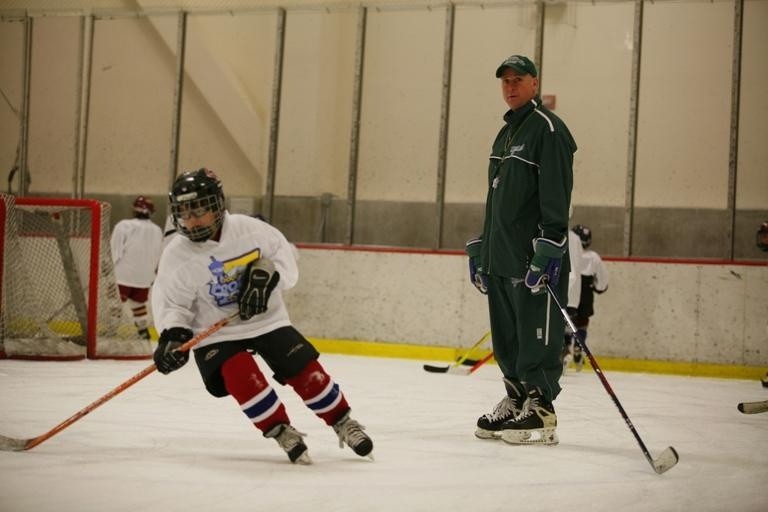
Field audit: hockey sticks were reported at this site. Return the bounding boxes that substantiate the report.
[0,308,239,452]
[737,400,767,413]
[423,328,496,376]
[540,279,679,475]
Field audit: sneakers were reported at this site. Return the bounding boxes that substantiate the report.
[472,376,527,431]
[137,329,152,341]
[261,421,314,465]
[500,385,561,430]
[330,406,378,463]
[573,346,586,373]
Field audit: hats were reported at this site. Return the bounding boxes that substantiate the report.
[495,55,537,78]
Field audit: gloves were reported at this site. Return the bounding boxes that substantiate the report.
[525,234,569,296]
[153,327,194,376]
[465,237,489,295]
[236,257,280,321]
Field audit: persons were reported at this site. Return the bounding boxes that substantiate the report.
[570,223,609,370]
[464,55,579,446]
[97,192,163,343]
[565,220,582,358]
[148,167,376,463]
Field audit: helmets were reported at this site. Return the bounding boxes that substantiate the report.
[571,224,592,250]
[755,221,768,252]
[131,194,155,218]
[168,167,225,243]
